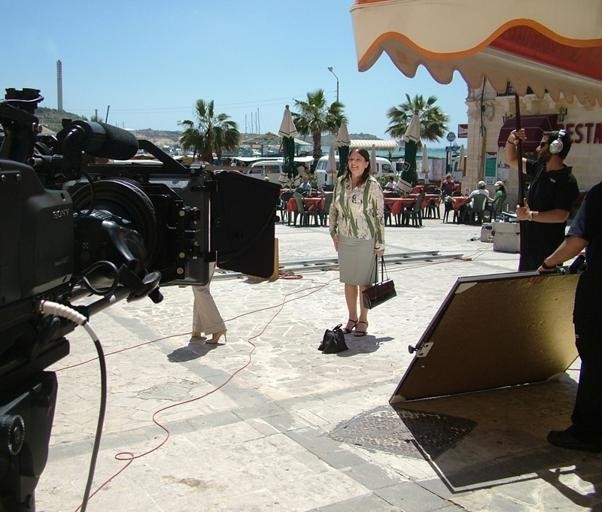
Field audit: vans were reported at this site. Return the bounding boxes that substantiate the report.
[137,155,397,190]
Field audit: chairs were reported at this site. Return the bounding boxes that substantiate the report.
[277,183,503,228]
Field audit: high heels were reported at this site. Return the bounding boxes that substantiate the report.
[354,320,368,337]
[206,328,227,344]
[192,329,201,337]
[342,319,358,334]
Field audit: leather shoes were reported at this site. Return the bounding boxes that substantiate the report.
[547,424,602,452]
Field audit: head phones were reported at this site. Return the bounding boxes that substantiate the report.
[549,129,567,155]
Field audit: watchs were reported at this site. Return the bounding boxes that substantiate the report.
[527,210,533,222]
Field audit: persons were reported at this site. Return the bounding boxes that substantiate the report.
[464,180,490,221]
[188,260,229,344]
[537,181,601,452]
[327,147,387,339]
[382,176,397,191]
[487,180,507,216]
[298,175,312,197]
[505,126,580,274]
[442,173,454,196]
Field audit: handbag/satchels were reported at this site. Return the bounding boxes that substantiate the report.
[362,247,396,310]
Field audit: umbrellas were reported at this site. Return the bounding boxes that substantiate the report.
[458,144,465,181]
[326,146,338,186]
[336,118,351,181]
[419,144,430,185]
[396,109,421,193]
[370,144,378,176]
[279,105,301,191]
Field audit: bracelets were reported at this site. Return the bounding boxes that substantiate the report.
[542,261,557,270]
[507,139,514,145]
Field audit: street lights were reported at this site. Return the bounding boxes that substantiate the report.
[328,66,339,102]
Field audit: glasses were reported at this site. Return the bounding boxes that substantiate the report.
[537,142,548,148]
